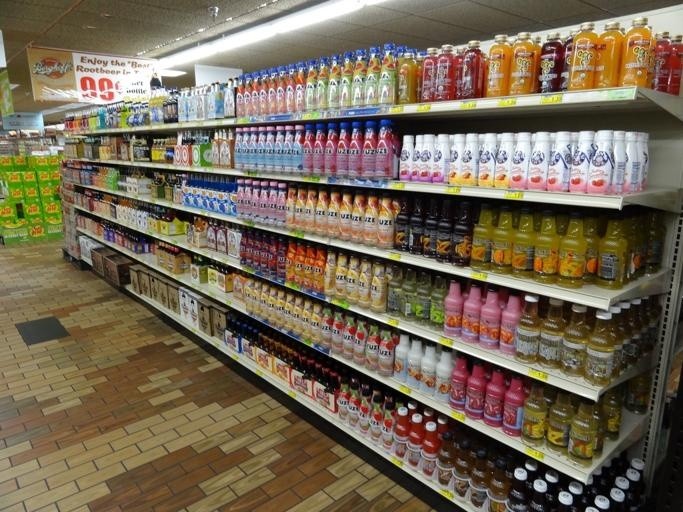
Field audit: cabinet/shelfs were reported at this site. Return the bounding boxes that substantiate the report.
[59,4,683,511]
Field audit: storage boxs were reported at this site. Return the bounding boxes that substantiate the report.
[79,236,134,286]
[0,154,63,249]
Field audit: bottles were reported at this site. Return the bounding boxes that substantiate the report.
[135,118,668,296]
[82,187,134,257]
[225,269,664,512]
[63,136,100,161]
[63,11,681,133]
